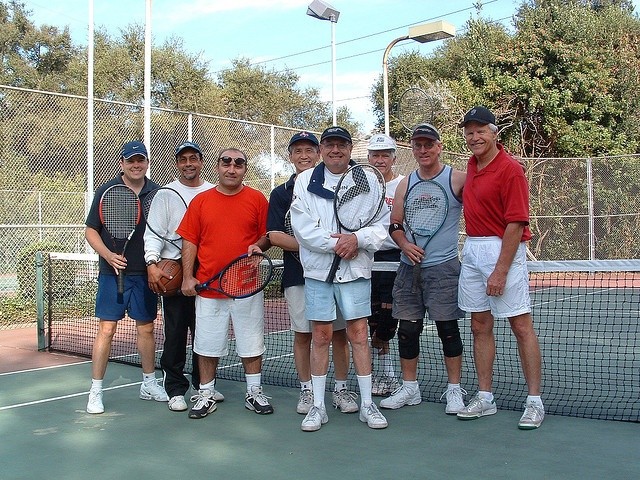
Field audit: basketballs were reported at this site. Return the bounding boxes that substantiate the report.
[151,259,183,297]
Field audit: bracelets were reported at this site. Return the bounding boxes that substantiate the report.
[387,221,405,236]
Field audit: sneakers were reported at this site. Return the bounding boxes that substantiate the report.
[87,387,104,413]
[188,389,217,418]
[300,405,328,431]
[457,392,497,420]
[379,380,422,410]
[331,387,359,414]
[244,385,274,415]
[359,401,388,429]
[440,385,467,415]
[189,386,225,402]
[518,398,545,430]
[372,375,400,395]
[139,378,169,401]
[297,387,314,413]
[168,396,187,411]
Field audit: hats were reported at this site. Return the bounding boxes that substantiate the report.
[367,135,397,151]
[320,126,352,143]
[459,107,495,128]
[175,142,203,156]
[411,125,440,141]
[288,132,319,151]
[120,141,148,160]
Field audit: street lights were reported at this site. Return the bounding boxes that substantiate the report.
[381,20,457,137]
[307,0,342,128]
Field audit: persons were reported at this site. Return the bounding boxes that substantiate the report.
[365,134,407,397]
[456,106,546,429]
[381,122,527,415]
[175,148,274,419]
[85,141,170,414]
[143,141,225,412]
[265,131,359,413]
[290,126,391,432]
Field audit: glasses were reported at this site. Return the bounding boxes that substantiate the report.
[322,142,350,149]
[219,156,246,165]
[178,155,198,163]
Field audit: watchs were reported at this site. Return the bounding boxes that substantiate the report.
[146,260,157,267]
